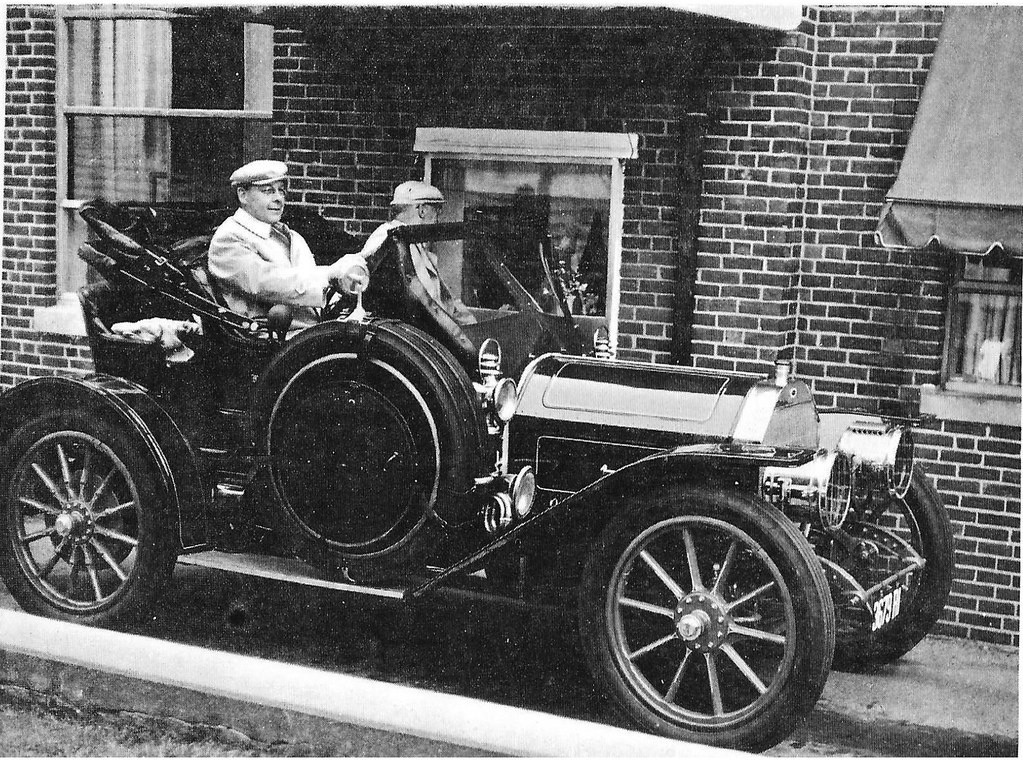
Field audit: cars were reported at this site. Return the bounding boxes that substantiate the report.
[0,197,955,753]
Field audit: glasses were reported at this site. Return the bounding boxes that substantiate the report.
[416,203,442,209]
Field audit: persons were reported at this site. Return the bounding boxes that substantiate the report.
[362,182,478,325]
[207,160,368,338]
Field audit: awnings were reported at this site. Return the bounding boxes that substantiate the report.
[874,6,1023,260]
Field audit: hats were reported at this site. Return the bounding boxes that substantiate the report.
[389,181,447,204]
[230,159,288,186]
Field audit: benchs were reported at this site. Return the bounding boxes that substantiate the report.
[75,281,223,417]
[126,202,360,342]
